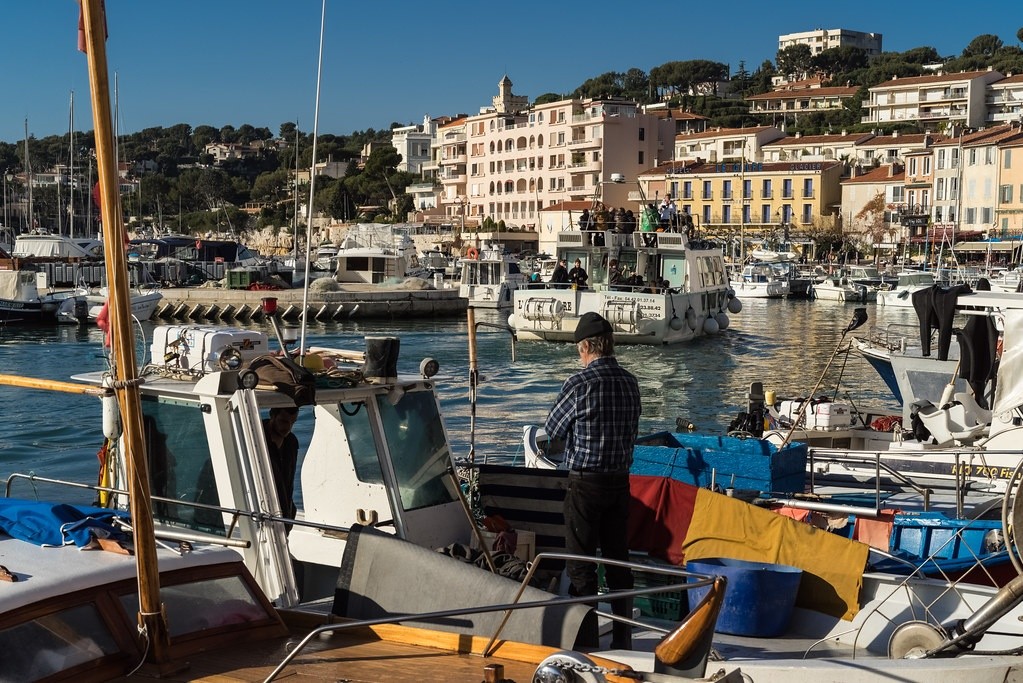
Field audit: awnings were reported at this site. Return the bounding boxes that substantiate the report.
[900,228,1023,266]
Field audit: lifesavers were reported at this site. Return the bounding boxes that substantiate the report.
[467,247,479,260]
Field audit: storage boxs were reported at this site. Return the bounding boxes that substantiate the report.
[591,550,691,621]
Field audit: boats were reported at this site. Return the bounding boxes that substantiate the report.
[75,319,1022,683]
[510,222,745,345]
[0,0,733,683]
[457,140,1022,438]
[0,64,342,326]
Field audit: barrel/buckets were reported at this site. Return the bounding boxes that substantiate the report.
[685,558,803,637]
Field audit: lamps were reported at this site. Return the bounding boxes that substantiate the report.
[419,357,440,380]
[236,369,260,388]
[214,344,243,373]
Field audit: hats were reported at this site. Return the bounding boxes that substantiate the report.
[574,311,613,343]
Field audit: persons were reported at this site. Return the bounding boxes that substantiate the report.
[527,193,695,294]
[817,247,856,264]
[544,311,642,650]
[892,255,897,265]
